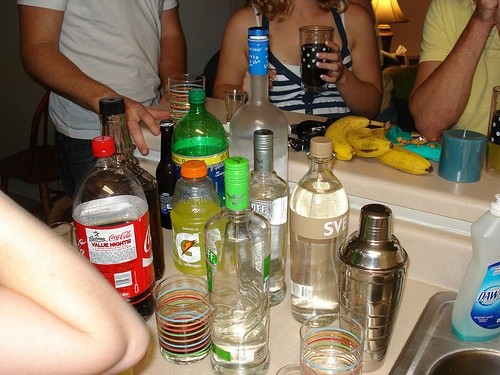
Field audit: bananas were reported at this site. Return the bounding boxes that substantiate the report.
[375,133,433,175]
[324,115,395,163]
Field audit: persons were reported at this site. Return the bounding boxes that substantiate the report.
[214,0,384,121]
[0,191,150,375]
[18,0,188,197]
[408,0,500,140]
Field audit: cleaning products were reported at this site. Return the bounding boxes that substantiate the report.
[452,193,500,342]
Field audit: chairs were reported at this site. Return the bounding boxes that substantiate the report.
[0,91,61,223]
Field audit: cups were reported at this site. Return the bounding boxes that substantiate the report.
[152,274,213,365]
[438,129,488,184]
[167,76,206,128]
[484,86,500,178]
[48,221,74,246]
[224,89,247,122]
[276,363,316,375]
[299,312,365,375]
[298,24,334,92]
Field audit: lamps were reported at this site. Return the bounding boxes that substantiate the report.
[371,0,409,52]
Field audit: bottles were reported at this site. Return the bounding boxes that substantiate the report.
[226,26,289,186]
[98,96,166,282]
[451,193,500,343]
[171,160,222,276]
[71,135,157,321]
[246,128,290,308]
[288,136,350,326]
[335,203,409,373]
[170,88,230,214]
[205,156,271,375]
[155,119,181,230]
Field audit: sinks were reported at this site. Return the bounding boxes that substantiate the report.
[389,291,500,375]
